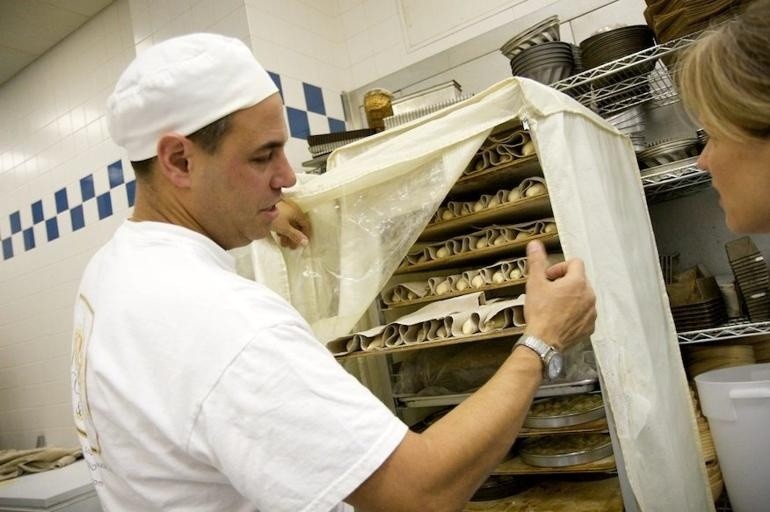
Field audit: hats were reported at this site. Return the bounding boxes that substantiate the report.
[105,33,280,162]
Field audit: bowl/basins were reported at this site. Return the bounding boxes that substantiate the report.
[503,14,574,84]
[611,104,707,169]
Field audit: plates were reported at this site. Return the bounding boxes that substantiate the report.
[582,25,652,69]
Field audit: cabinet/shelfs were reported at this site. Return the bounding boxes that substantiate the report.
[323,20,766,510]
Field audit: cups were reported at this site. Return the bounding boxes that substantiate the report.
[363,89,394,128]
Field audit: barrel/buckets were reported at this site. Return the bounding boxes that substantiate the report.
[692,363,770,512]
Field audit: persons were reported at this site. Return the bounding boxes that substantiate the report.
[71,32,597,512]
[667,1,770,237]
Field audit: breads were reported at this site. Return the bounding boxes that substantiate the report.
[517,393,610,455]
[347,141,555,350]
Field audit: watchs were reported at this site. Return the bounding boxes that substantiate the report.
[512,334,564,382]
